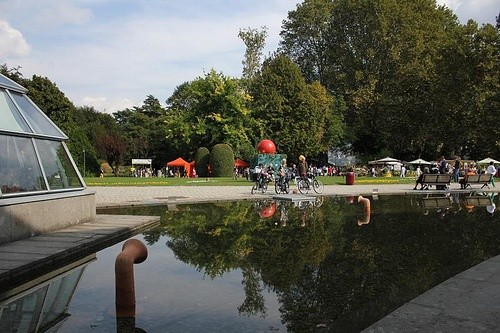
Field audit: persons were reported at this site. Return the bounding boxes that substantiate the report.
[419,194,496,217]
[1,171,28,193]
[233,157,406,194]
[193,167,197,177]
[299,207,307,228]
[280,202,289,228]
[413,155,500,190]
[99,166,106,178]
[132,166,189,179]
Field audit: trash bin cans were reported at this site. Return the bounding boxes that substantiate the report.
[346,172,354,185]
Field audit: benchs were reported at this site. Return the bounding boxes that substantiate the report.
[422,194,451,209]
[466,192,492,207]
[417,174,454,191]
[461,174,492,190]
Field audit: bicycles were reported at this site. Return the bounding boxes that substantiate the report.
[251,173,270,195]
[297,175,325,195]
[274,176,291,194]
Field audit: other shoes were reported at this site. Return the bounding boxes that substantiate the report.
[424,188,428,190]
[461,188,463,189]
[420,188,423,190]
[413,188,417,190]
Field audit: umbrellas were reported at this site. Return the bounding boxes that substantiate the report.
[408,158,432,164]
[377,157,400,161]
[479,192,498,196]
[477,157,500,164]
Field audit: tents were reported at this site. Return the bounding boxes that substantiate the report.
[167,157,190,178]
[234,159,251,167]
